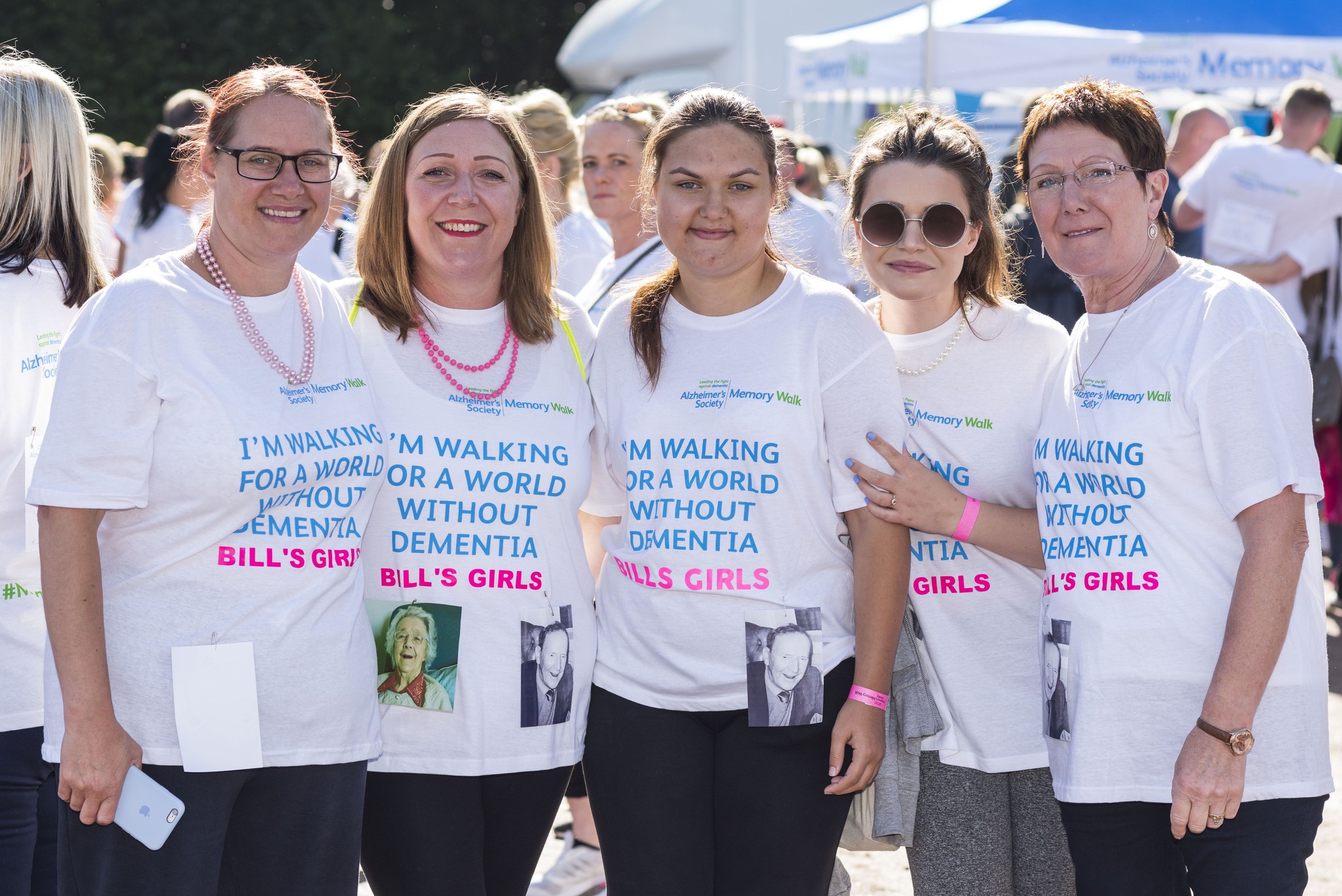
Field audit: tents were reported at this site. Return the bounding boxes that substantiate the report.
[790,0,1342,180]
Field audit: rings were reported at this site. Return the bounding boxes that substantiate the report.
[1208,812,1225,824]
[889,494,898,507]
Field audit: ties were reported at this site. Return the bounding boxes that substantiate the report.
[546,690,555,702]
[1045,699,1053,720]
[778,692,790,704]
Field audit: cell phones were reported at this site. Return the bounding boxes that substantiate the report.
[113,766,186,851]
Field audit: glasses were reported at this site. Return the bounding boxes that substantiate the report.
[215,143,342,183]
[1023,161,1149,195]
[393,631,429,645]
[858,201,977,250]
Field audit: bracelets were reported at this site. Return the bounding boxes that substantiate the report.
[847,684,889,709]
[952,494,982,543]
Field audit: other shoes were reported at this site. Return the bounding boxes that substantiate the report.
[524,828,605,896]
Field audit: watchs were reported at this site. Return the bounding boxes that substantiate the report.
[1197,716,1255,755]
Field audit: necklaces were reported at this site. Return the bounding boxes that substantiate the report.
[1069,247,1169,394]
[410,317,521,398]
[196,225,317,390]
[870,298,976,377]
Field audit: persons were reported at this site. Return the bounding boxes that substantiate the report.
[0,44,1341,896]
